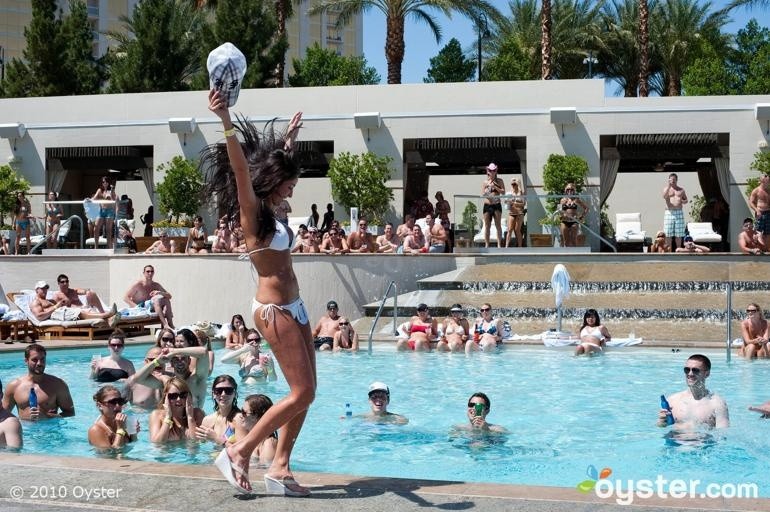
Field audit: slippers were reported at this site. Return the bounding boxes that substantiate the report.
[263,473,311,497]
[214,447,254,495]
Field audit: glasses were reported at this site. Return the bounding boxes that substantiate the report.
[247,338,260,343]
[60,280,69,283]
[467,401,488,407]
[310,231,316,234]
[684,366,708,374]
[161,336,174,342]
[330,233,338,236]
[110,343,123,348]
[167,391,187,399]
[360,224,366,226]
[480,308,490,312]
[339,322,349,326]
[746,309,758,312]
[100,397,127,405]
[148,358,155,361]
[333,225,339,227]
[213,386,235,394]
[241,407,254,417]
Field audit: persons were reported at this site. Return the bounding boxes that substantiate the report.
[675,234,711,253]
[1,345,76,420]
[90,329,136,381]
[123,347,167,409]
[11,193,32,257]
[1,235,7,254]
[185,216,210,255]
[222,329,279,386]
[342,383,408,424]
[144,327,175,367]
[231,226,248,253]
[506,176,527,246]
[149,375,204,444]
[664,173,689,251]
[84,176,119,249]
[185,374,248,449]
[451,391,508,436]
[229,394,278,464]
[748,173,770,235]
[435,304,470,353]
[145,232,179,254]
[30,282,118,321]
[739,306,769,359]
[53,273,109,313]
[658,353,730,433]
[1,381,25,450]
[560,183,589,248]
[312,300,355,351]
[334,319,359,353]
[194,41,318,498]
[228,313,248,349]
[175,328,200,348]
[88,384,143,451]
[481,162,504,250]
[293,190,452,253]
[576,309,611,355]
[211,217,232,253]
[464,301,508,354]
[158,347,210,409]
[45,191,64,249]
[124,264,176,331]
[649,232,668,253]
[193,330,215,374]
[740,217,765,254]
[395,303,439,352]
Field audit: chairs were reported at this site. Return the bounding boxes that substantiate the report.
[6,291,167,341]
[8,283,154,340]
[687,222,723,253]
[613,213,653,253]
[85,218,135,249]
[0,318,28,343]
[18,220,71,255]
[473,218,507,247]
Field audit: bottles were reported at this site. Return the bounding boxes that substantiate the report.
[475,403,482,416]
[199,227,204,238]
[685,243,693,249]
[473,324,478,343]
[655,238,664,245]
[27,387,37,408]
[147,301,153,314]
[660,394,675,426]
[344,404,352,421]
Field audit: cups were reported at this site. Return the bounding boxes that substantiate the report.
[126,416,137,434]
[260,353,270,369]
[93,353,101,367]
[425,328,432,337]
[474,331,480,344]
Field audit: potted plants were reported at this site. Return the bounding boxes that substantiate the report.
[149,155,209,237]
[0,164,33,254]
[746,140,765,251]
[326,151,396,237]
[530,151,592,247]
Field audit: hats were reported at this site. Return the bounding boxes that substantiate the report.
[683,235,693,241]
[367,382,390,397]
[417,303,428,311]
[205,41,247,109]
[450,304,463,311]
[34,281,50,289]
[485,163,498,170]
[327,300,338,310]
